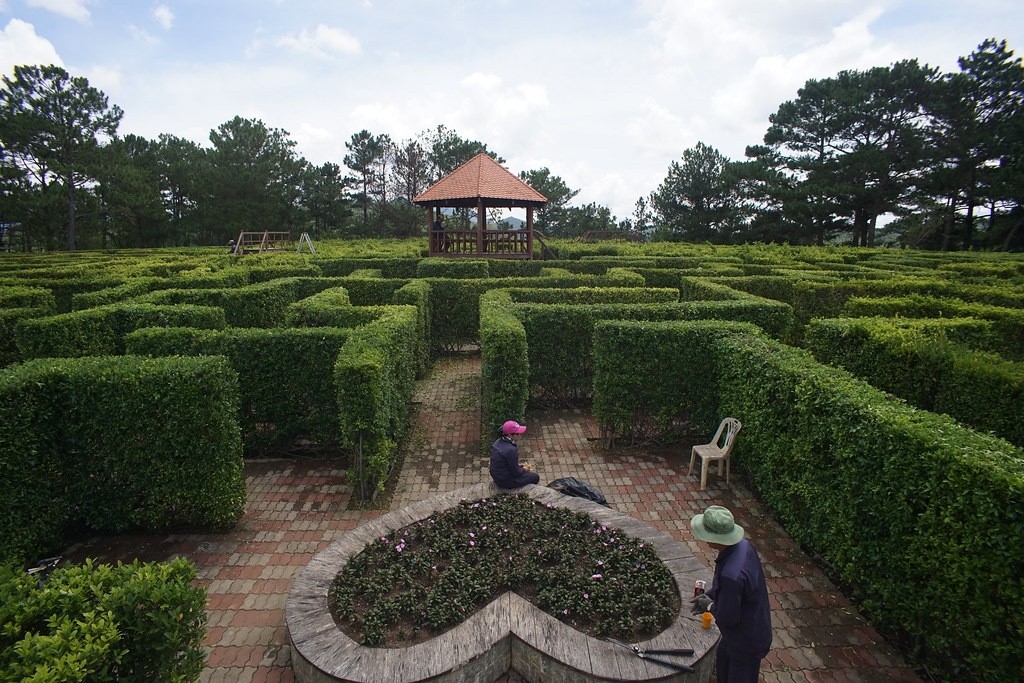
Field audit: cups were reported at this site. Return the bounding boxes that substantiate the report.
[703,612,712,629]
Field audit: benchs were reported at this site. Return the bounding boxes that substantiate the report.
[433,238,529,254]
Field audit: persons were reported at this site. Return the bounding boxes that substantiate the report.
[689,506,773,683]
[227,240,237,255]
[490,421,539,488]
[434,215,450,252]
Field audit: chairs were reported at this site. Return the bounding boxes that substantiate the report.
[688,417,742,491]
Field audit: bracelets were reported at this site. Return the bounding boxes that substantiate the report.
[707,602,713,611]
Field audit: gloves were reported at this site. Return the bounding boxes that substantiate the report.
[689,593,714,616]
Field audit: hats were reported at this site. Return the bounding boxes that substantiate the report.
[502,421,526,435]
[690,505,745,545]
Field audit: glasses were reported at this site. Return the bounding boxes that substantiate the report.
[512,433,523,435]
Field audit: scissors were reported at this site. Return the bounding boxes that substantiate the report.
[606,636,694,674]
